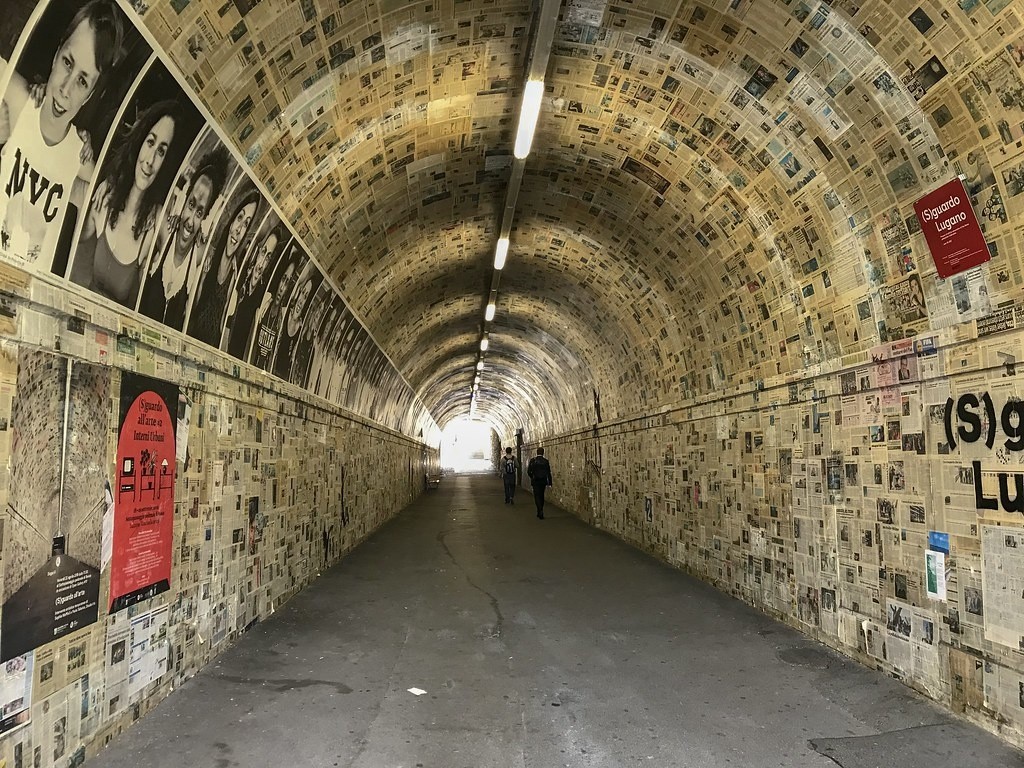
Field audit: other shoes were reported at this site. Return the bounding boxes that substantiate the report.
[537,515,543,519]
[505,499,514,505]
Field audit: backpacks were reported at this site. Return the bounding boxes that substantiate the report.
[504,455,516,474]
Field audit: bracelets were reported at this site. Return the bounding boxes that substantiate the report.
[917,304,920,308]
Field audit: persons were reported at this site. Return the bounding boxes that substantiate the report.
[646,499,652,522]
[138,147,229,331]
[501,447,519,504]
[79,99,186,308]
[898,356,911,381]
[721,303,732,315]
[909,275,928,317]
[318,315,350,398]
[967,146,989,182]
[0,0,124,274]
[306,302,342,393]
[224,226,283,360]
[291,288,333,388]
[272,276,316,381]
[967,591,982,614]
[186,191,260,348]
[249,250,302,371]
[875,465,882,483]
[528,448,552,519]
[330,325,405,427]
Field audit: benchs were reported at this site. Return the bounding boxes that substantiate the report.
[424,473,439,487]
